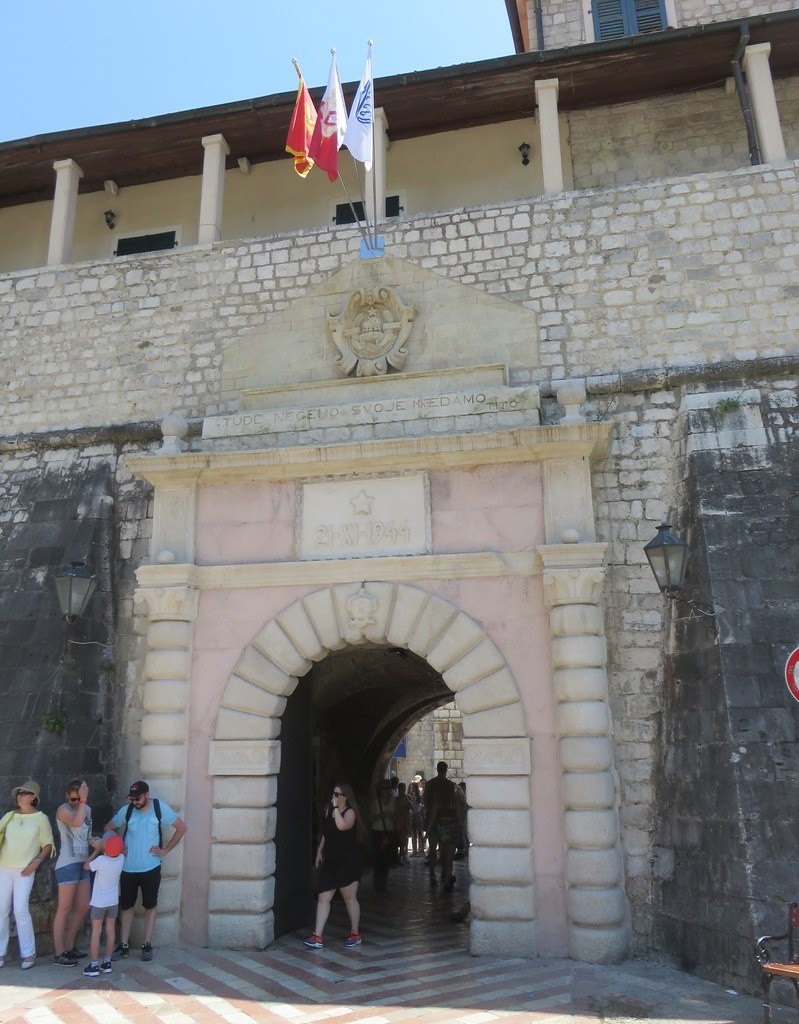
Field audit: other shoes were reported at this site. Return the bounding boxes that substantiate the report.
[416,852,425,857]
[444,876,456,891]
[0,960,5,968]
[22,954,36,969]
[410,851,419,856]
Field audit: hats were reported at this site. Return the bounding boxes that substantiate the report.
[102,831,124,856]
[413,775,422,783]
[12,781,40,804]
[125,781,149,798]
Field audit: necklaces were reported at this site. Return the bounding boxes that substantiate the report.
[18,806,34,825]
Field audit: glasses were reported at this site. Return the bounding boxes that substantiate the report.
[16,791,34,796]
[68,794,80,801]
[129,793,145,801]
[332,792,344,797]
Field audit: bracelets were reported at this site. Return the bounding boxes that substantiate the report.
[79,801,88,804]
[334,806,339,809]
[35,857,44,864]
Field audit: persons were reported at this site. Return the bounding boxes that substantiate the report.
[369,761,468,890]
[83,830,125,977]
[53,778,91,967]
[0,781,57,970]
[303,781,369,948]
[104,781,188,961]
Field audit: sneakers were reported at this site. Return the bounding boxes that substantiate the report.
[99,960,112,973]
[141,942,153,960]
[304,932,326,948]
[345,930,361,946]
[83,963,99,976]
[53,951,77,966]
[71,947,88,958]
[111,943,129,961]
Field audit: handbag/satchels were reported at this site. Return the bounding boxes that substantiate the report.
[370,830,392,856]
[0,810,15,847]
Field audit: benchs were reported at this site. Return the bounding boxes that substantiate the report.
[753,901,799,1024]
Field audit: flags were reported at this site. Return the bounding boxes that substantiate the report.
[281,55,373,182]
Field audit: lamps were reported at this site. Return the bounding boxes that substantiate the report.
[518,141,531,166]
[54,559,98,626]
[104,209,116,230]
[643,517,714,620]
